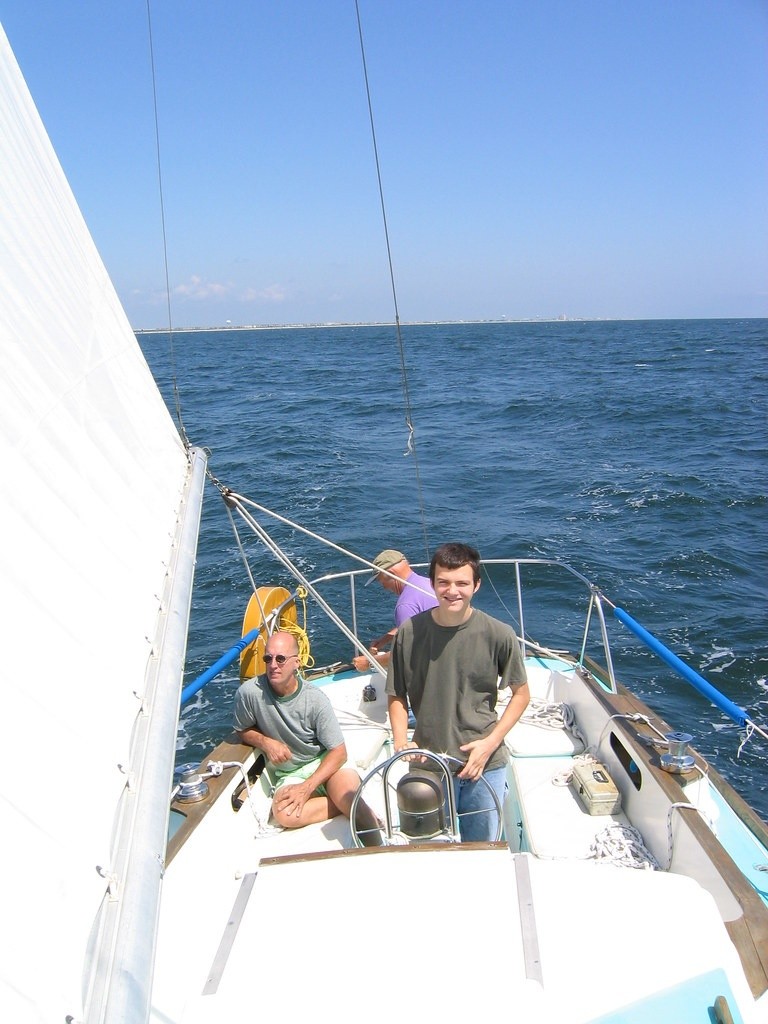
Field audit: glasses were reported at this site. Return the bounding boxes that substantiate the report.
[262,654,299,664]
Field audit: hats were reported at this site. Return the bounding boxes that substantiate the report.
[364,549,407,586]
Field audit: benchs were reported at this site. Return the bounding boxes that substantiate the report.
[246,705,656,868]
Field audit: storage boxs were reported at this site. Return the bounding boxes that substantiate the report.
[572,763,622,816]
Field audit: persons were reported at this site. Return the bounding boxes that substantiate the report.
[232,632,387,847]
[353,549,440,727]
[385,543,530,842]
